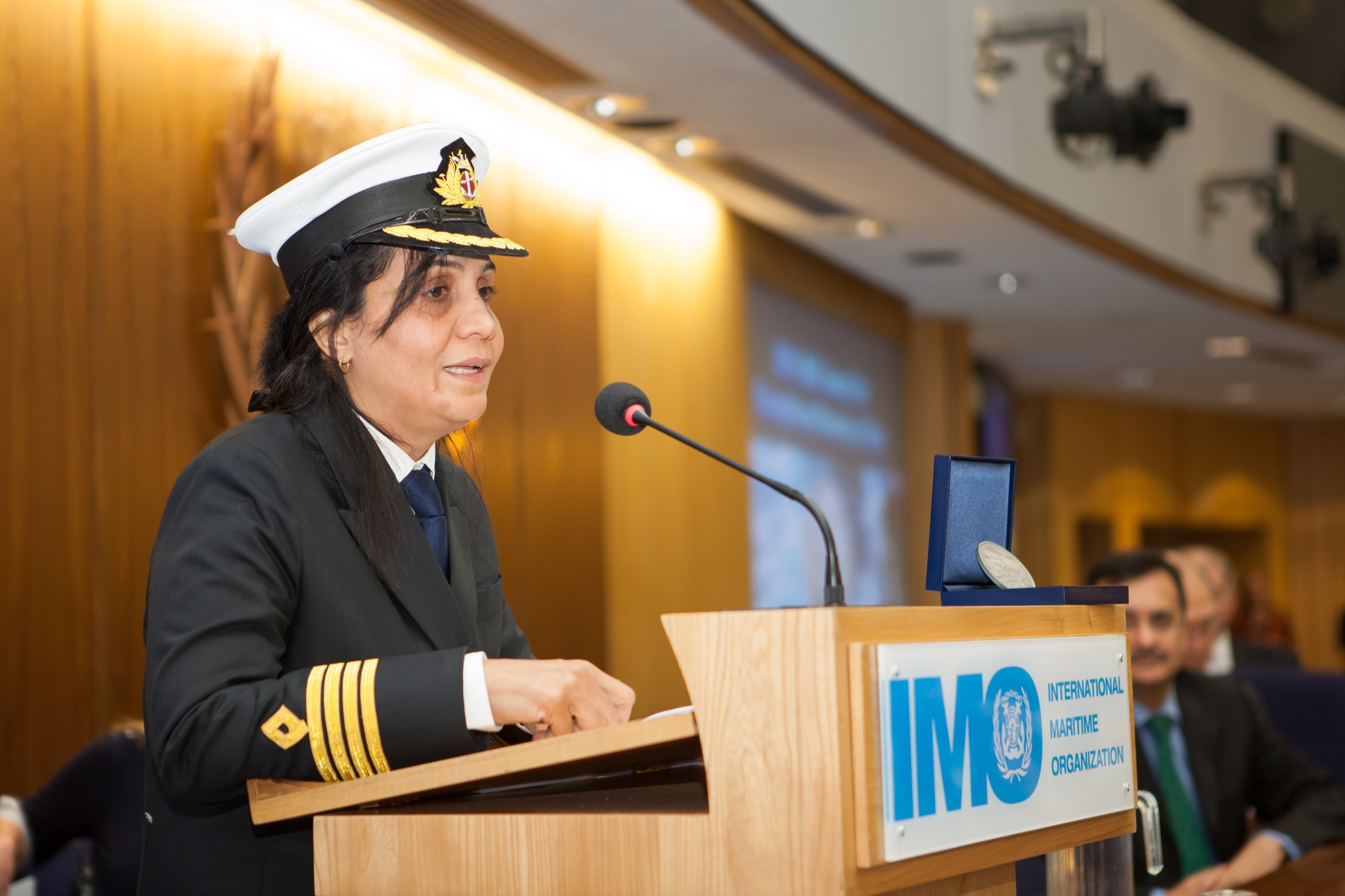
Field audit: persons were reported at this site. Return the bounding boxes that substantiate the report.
[0,715,147,896]
[1176,541,1302,683]
[139,116,639,896]
[1014,551,1345,896]
[1144,548,1225,680]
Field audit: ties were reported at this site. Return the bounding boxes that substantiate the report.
[1145,712,1214,880]
[398,463,449,584]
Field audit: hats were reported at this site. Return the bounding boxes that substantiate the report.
[234,122,529,292]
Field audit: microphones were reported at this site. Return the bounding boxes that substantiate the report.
[594,380,850,606]
